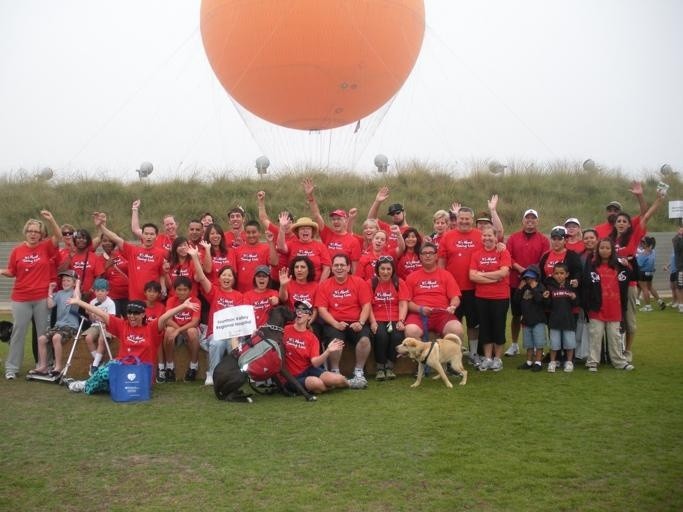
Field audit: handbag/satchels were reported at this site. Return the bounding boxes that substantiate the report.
[108,355,151,402]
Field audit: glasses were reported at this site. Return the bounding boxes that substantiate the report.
[419,251,435,255]
[390,210,400,216]
[62,231,73,236]
[293,306,310,313]
[377,255,393,265]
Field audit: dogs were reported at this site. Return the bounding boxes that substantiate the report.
[212,305,318,404]
[395,333,468,388]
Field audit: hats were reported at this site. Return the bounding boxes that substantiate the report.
[475,216,492,224]
[524,209,580,239]
[293,300,312,309]
[255,266,269,276]
[606,202,622,210]
[290,217,318,235]
[522,271,537,278]
[329,209,346,217]
[126,301,145,312]
[387,204,403,215]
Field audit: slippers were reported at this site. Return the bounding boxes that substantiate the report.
[50,371,59,375]
[26,368,48,375]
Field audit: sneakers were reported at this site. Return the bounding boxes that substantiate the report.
[165,368,176,383]
[68,380,86,393]
[182,367,196,383]
[90,364,97,376]
[516,346,634,373]
[98,359,105,368]
[330,369,425,389]
[634,298,683,313]
[155,367,167,383]
[467,353,503,371]
[504,345,520,356]
[4,372,15,380]
[203,370,216,386]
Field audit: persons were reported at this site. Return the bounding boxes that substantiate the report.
[0,176,683,394]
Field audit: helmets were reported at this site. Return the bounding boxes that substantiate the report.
[58,270,75,278]
